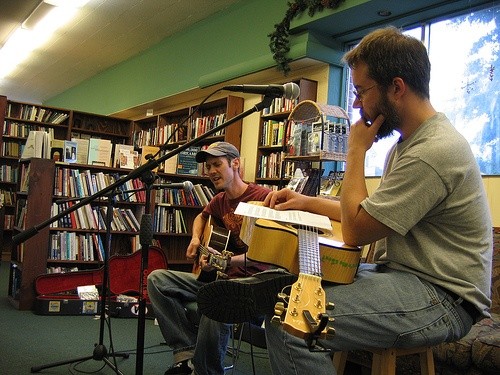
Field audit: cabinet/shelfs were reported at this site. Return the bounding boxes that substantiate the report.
[0,78,349,310]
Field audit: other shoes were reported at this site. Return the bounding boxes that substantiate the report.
[197,269,298,324]
[164,359,194,375]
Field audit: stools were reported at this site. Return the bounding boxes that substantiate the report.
[333,344,437,375]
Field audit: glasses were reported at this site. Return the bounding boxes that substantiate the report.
[353,84,381,100]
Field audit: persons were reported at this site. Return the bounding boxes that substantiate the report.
[145,139,282,374]
[196,24,495,374]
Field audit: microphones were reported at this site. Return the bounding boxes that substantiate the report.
[152,180,193,192]
[223,81,301,100]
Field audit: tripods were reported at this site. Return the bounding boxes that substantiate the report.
[30,181,154,375]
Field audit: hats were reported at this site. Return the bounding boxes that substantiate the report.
[195,141,239,163]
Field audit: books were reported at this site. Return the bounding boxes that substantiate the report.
[1,88,297,274]
